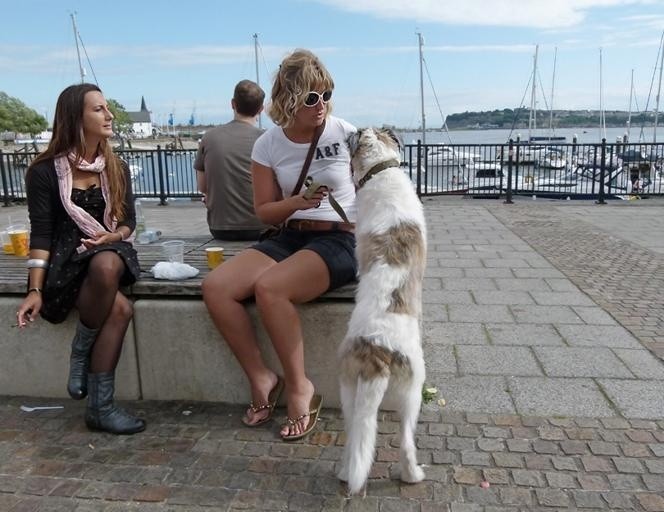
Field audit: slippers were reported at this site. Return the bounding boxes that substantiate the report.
[241,375,285,427]
[281,395,323,440]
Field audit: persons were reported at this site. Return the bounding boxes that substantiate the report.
[193,80,282,243]
[201,47,362,440]
[16,83,150,437]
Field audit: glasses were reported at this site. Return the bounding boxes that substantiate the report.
[303,90,332,107]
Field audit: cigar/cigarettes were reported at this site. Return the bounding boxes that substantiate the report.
[10,321,27,327]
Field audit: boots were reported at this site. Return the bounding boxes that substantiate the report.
[67,320,101,398]
[84,369,146,434]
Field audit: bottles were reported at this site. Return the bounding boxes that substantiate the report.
[132,201,161,245]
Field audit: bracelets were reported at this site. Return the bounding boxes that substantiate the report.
[27,256,51,269]
[116,229,124,241]
[27,285,43,295]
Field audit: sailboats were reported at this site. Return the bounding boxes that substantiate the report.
[408,32,482,169]
[465,25,664,197]
[70,10,144,183]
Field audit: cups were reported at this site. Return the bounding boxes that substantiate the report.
[159,240,184,263]
[0,223,29,257]
[204,247,223,270]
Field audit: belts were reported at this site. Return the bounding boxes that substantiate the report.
[286,220,356,233]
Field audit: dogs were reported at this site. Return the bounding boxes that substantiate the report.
[337,125,425,493]
